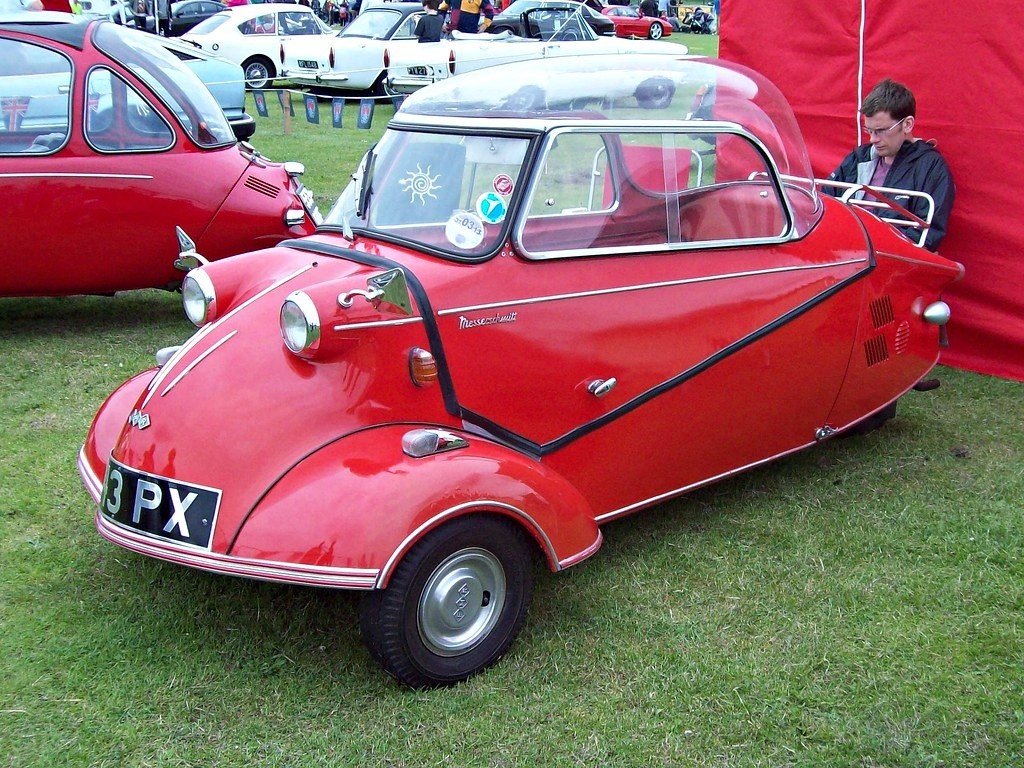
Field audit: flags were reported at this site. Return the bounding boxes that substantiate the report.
[250,89,404,129]
[89,92,100,119]
[0,95,31,132]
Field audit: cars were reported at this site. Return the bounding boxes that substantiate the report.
[76,55,967,691]
[161,1,689,117]
[0,10,324,298]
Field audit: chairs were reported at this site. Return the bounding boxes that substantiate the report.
[560,144,702,214]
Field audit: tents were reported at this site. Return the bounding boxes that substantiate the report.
[716,1,1023,385]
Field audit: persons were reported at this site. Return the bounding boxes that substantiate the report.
[152,0,173,38]
[413,0,494,44]
[132,0,151,28]
[71,1,82,16]
[820,78,956,256]
[640,0,718,33]
[300,0,385,26]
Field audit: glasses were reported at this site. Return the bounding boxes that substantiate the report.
[862,117,906,136]
